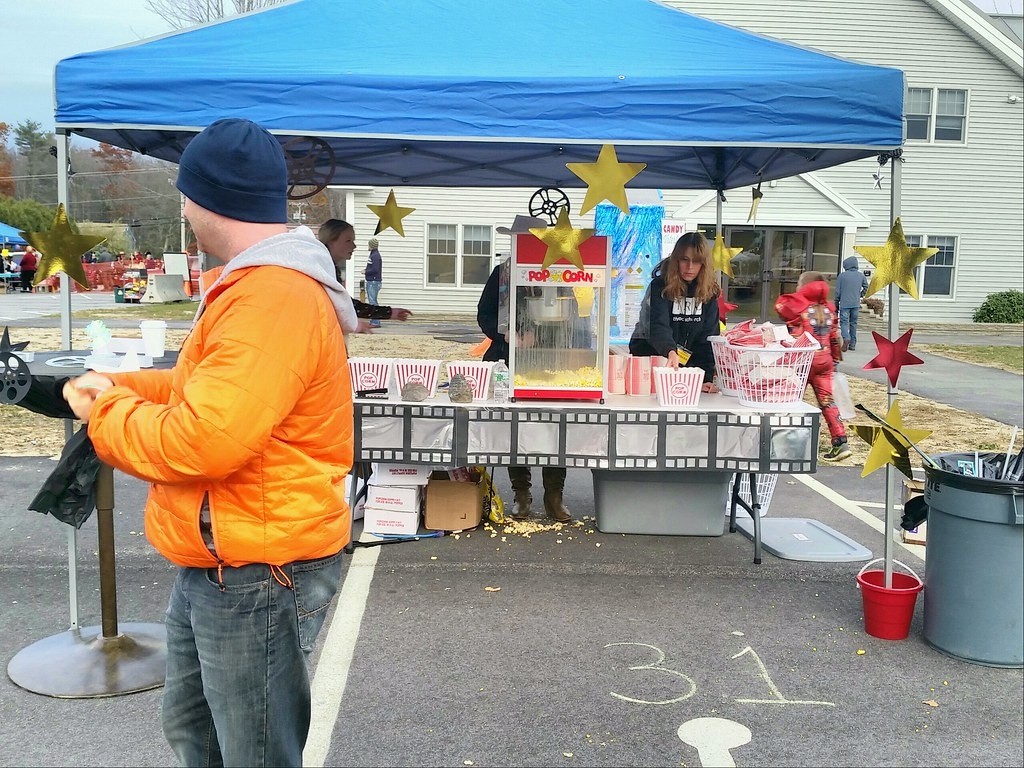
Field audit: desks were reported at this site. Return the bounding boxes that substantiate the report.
[355,386,823,563]
[0,273,20,293]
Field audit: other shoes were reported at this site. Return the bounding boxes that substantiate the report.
[21,290,32,293]
[849,345,855,350]
[368,324,382,328]
[842,339,850,351]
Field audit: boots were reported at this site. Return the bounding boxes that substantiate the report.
[542,466,572,521]
[508,466,533,519]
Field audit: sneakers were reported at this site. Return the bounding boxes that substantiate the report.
[824,442,853,462]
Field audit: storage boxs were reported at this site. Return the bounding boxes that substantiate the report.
[348,355,705,405]
[363,485,422,512]
[364,509,421,536]
[425,470,486,532]
[114,287,131,303]
[590,470,732,537]
[367,462,433,487]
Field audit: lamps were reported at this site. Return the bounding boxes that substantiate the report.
[770,180,776,187]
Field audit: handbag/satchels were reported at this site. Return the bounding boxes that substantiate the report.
[468,465,505,524]
[831,371,857,419]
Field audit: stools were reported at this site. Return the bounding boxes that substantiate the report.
[35,285,49,293]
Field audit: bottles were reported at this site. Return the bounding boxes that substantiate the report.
[494,359,509,404]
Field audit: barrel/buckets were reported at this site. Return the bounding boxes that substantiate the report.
[856,558,925,641]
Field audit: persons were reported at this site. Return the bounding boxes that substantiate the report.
[62,120,361,768]
[81,247,154,269]
[318,220,413,322]
[478,214,592,521]
[0,245,43,292]
[362,238,382,327]
[629,233,720,394]
[834,257,869,352]
[788,271,853,462]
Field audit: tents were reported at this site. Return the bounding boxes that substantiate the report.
[52,0,906,635]
[0,222,30,293]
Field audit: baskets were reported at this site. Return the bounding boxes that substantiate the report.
[706,330,816,410]
[724,471,778,517]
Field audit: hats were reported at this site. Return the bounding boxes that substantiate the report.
[175,118,288,224]
[368,239,378,249]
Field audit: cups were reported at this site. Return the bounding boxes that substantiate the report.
[139,319,167,358]
[608,355,668,396]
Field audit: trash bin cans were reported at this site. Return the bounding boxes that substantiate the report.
[920,450,1024,671]
